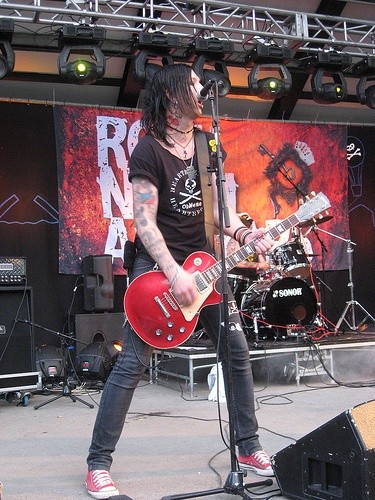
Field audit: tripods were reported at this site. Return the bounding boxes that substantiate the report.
[161,93,274,500]
[315,227,375,335]
[32,321,95,409]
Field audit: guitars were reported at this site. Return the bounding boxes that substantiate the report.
[124,191,332,349]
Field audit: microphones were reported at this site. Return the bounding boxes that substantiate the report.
[196,78,216,101]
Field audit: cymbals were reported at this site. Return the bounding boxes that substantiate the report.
[304,253,321,257]
[215,214,254,235]
[295,215,333,228]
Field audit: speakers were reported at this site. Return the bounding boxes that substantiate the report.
[269,399,375,500]
[82,254,114,311]
[0,284,36,375]
[70,313,126,379]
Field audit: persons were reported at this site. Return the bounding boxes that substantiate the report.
[85,63,275,499]
[226,215,269,310]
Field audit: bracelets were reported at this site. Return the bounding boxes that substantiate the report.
[170,266,182,289]
[234,227,252,245]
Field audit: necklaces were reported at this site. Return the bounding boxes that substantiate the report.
[165,124,197,180]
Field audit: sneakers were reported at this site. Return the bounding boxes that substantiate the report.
[85,470,119,499]
[236,450,275,476]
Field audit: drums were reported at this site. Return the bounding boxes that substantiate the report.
[269,240,312,279]
[227,274,250,309]
[259,270,282,280]
[241,279,319,340]
